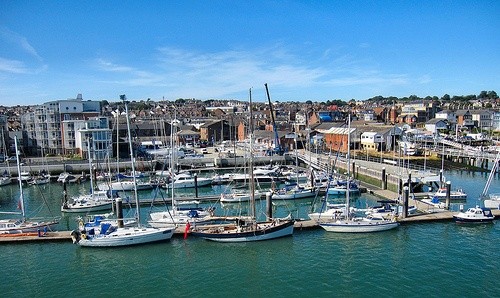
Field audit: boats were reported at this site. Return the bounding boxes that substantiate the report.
[432,181,467,200]
[452,206,496,223]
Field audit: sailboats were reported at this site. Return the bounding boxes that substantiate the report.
[0,83,419,247]
